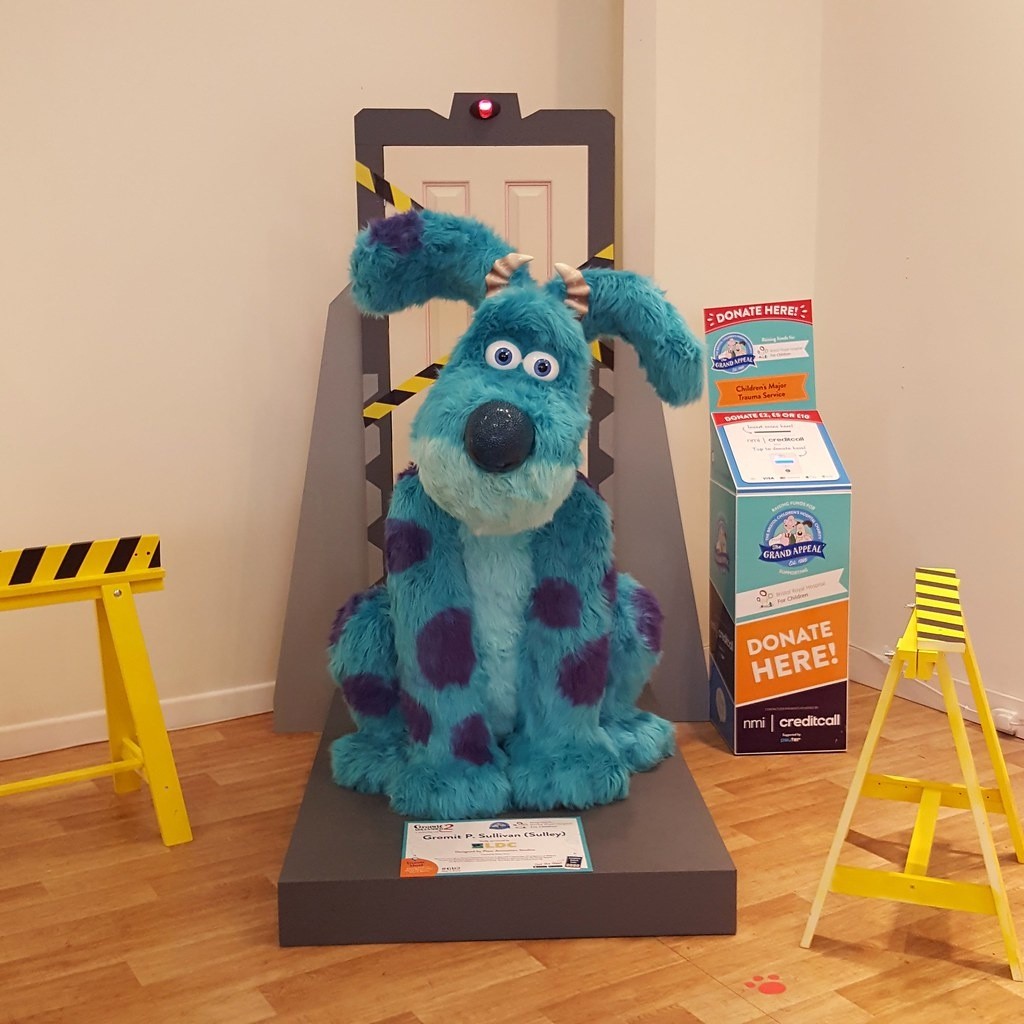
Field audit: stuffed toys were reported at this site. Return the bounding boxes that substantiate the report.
[326,207,702,823]
